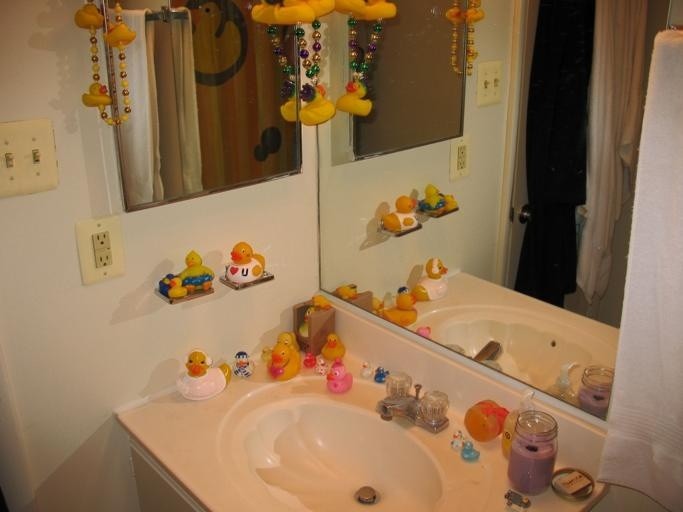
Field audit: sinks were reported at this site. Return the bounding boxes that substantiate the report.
[409,303,594,409]
[227,394,448,512]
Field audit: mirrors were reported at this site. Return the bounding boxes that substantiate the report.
[320,0,683,421]
[101,0,302,213]
[352,1,468,162]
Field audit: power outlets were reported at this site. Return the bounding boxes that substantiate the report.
[74,215,126,287]
[449,138,469,181]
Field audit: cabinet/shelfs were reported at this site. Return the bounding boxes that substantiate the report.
[129,444,200,512]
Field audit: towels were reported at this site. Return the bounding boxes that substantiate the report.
[514,0,596,308]
[169,6,204,195]
[109,8,165,208]
[575,0,650,307]
[599,30,683,512]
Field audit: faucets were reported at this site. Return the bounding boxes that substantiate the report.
[472,341,503,364]
[377,394,416,421]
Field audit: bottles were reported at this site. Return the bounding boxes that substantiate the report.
[577,364,614,421]
[507,409,558,496]
